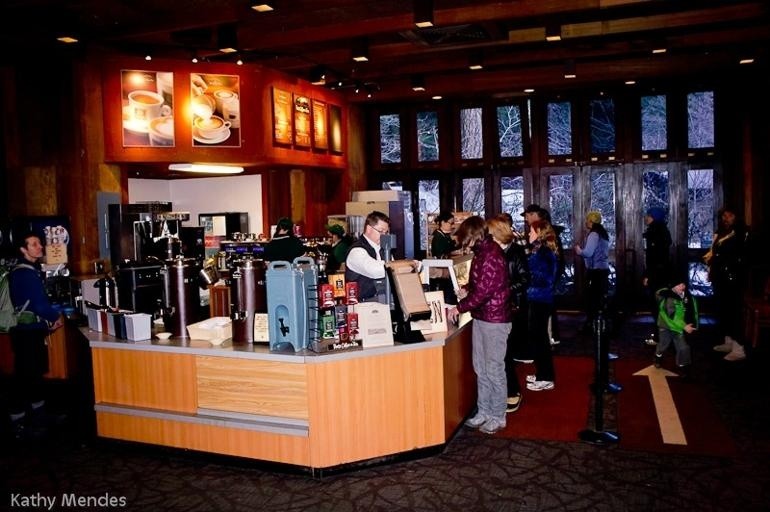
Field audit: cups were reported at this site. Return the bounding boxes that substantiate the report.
[156,73,173,109]
[149,116,173,147]
[127,89,171,127]
[213,90,238,113]
[223,100,240,128]
[194,93,216,118]
[195,115,232,139]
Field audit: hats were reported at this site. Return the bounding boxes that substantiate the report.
[325,224,344,235]
[587,212,601,224]
[520,204,539,216]
[648,208,668,220]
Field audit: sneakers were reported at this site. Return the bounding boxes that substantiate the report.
[526,375,555,391]
[654,353,663,368]
[466,413,484,429]
[479,419,506,434]
[505,393,522,413]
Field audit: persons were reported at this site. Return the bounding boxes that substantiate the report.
[7,232,64,421]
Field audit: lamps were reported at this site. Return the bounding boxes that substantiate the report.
[216,0,670,99]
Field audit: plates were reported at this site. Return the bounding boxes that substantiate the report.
[122,106,149,137]
[194,126,231,145]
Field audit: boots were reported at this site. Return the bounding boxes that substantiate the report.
[714,336,746,361]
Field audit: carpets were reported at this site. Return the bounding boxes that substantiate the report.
[488,350,740,459]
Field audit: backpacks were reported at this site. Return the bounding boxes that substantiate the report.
[1,264,35,334]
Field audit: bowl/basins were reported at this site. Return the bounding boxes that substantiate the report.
[194,264,220,291]
